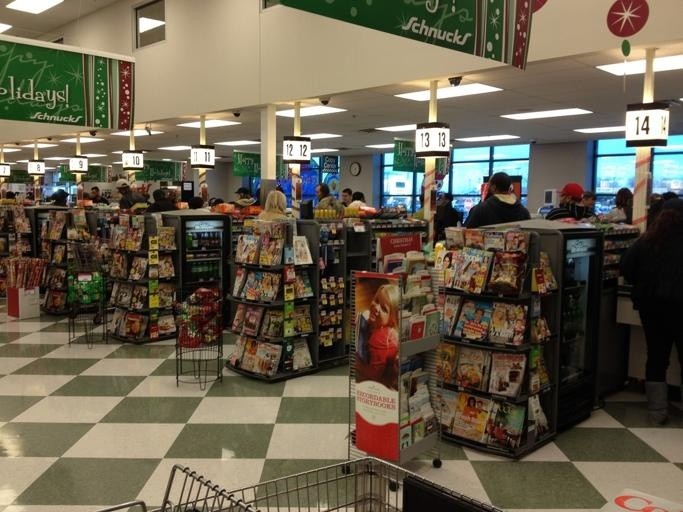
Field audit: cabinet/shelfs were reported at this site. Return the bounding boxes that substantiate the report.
[107,213,180,343]
[225,212,429,383]
[163,213,229,327]
[347,265,442,487]
[0,205,145,314]
[226,217,256,294]
[425,215,639,461]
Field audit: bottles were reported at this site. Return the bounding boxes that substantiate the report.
[185,231,221,280]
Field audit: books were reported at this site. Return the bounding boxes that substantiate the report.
[0,204,178,340]
[231,218,313,377]
[376,225,529,450]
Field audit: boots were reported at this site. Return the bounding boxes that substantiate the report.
[646,380,670,428]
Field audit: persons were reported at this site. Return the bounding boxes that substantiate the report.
[51,188,69,205]
[115,179,147,208]
[188,184,367,218]
[354,284,399,390]
[437,172,677,224]
[620,196,683,426]
[89,187,109,205]
[147,190,180,212]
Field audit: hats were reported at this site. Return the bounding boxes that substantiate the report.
[556,182,585,202]
[115,179,130,188]
[235,188,250,194]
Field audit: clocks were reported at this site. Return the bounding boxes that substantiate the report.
[350,162,361,176]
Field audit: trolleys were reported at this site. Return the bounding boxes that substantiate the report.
[101,454,499,512]
